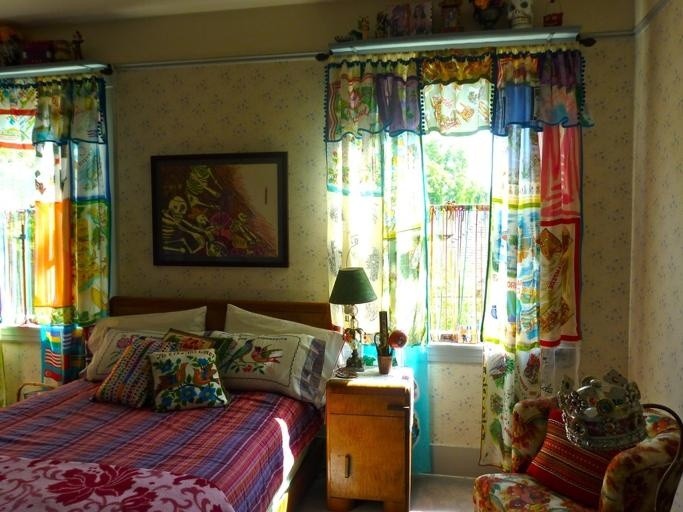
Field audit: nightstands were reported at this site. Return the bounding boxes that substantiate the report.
[326,364,412,511]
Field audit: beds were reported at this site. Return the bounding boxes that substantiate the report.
[0,294,333,512]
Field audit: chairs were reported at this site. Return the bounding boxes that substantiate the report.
[471,393,682,512]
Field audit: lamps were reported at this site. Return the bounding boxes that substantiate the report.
[329,265,377,373]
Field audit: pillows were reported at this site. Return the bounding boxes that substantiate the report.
[525,406,635,507]
[83,302,345,414]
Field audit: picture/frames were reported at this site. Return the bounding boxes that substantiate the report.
[148,149,290,270]
